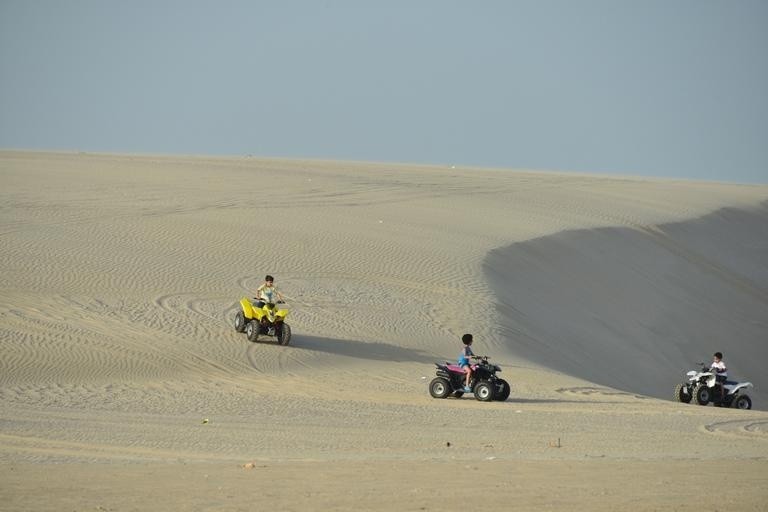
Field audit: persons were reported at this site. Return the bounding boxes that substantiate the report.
[458,334,480,393]
[256,275,283,304]
[708,352,728,400]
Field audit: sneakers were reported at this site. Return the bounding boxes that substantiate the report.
[464,386,472,392]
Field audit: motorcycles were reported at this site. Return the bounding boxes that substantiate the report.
[234,297,291,346]
[430,356,510,402]
[674,363,754,410]
